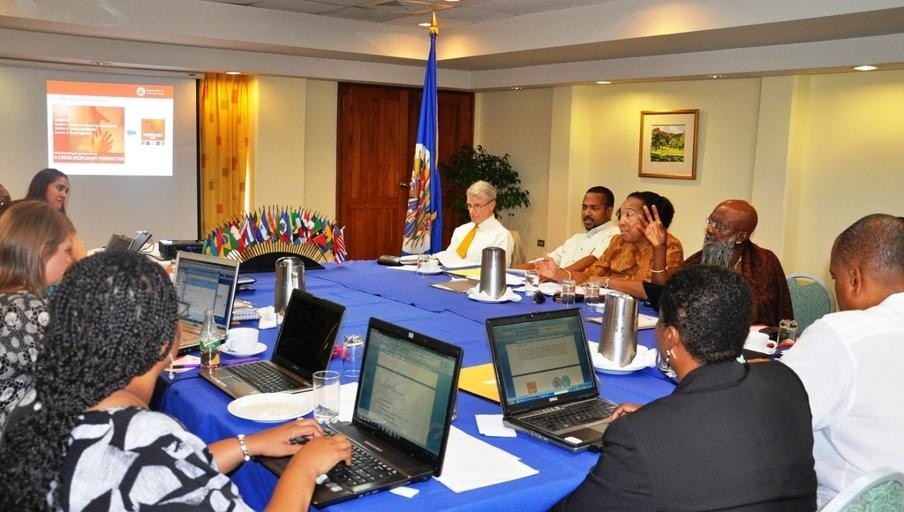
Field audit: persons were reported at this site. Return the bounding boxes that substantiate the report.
[511,185,621,285]
[0,250,353,512]
[0,198,77,439]
[544,263,818,512]
[443,179,515,269]
[635,199,794,343]
[27,167,89,261]
[776,213,904,512]
[531,190,683,303]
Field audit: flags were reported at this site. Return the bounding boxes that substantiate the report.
[399,23,444,258]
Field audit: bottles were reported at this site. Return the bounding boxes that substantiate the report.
[198,309,221,368]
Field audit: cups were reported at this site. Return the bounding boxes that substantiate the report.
[416,247,671,376]
[772,319,797,351]
[271,254,306,333]
[341,332,365,377]
[313,370,342,424]
[450,387,459,421]
[224,326,260,351]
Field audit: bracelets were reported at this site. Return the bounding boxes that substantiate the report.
[236,434,252,465]
[565,269,574,283]
[646,263,670,273]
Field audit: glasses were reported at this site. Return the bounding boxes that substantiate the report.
[462,200,495,209]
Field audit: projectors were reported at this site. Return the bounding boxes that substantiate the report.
[159,239,204,260]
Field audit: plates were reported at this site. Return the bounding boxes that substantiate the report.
[223,392,317,425]
[214,339,266,359]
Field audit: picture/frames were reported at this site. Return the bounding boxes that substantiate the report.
[636,108,701,181]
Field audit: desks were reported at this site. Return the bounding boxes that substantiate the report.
[79,253,800,511]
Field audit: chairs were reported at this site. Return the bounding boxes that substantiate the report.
[818,465,900,512]
[782,271,837,335]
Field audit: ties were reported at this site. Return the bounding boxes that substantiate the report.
[457,224,482,259]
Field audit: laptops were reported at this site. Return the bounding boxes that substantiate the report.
[111,233,136,250]
[251,316,464,510]
[167,250,241,357]
[197,288,346,399]
[128,231,152,253]
[486,307,633,454]
[642,281,665,313]
